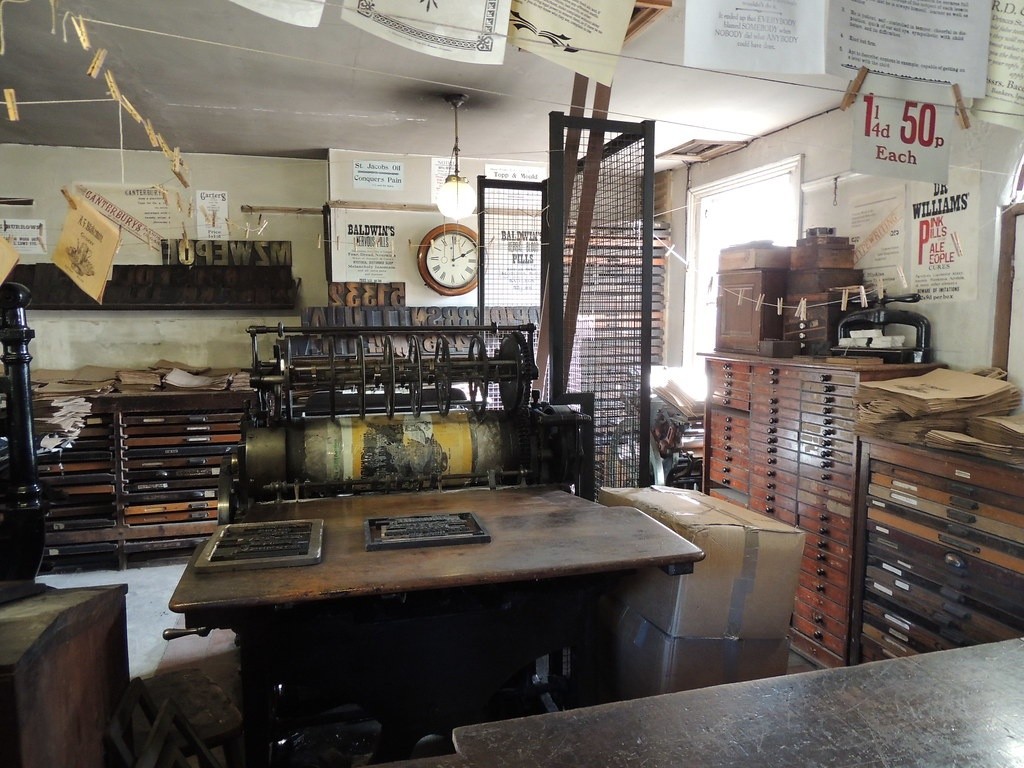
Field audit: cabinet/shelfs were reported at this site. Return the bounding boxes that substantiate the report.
[1,583,130,768]
[698,352,948,669]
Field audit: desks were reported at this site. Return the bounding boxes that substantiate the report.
[451,635,1024,767]
[168,490,705,767]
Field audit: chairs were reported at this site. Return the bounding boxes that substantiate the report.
[133,699,222,768]
[105,678,191,767]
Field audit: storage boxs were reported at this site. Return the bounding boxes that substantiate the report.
[602,485,807,638]
[616,601,791,704]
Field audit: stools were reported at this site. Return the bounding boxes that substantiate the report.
[144,667,245,766]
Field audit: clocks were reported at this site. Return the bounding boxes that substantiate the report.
[417,223,480,296]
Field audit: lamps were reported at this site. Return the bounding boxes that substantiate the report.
[437,93,478,221]
